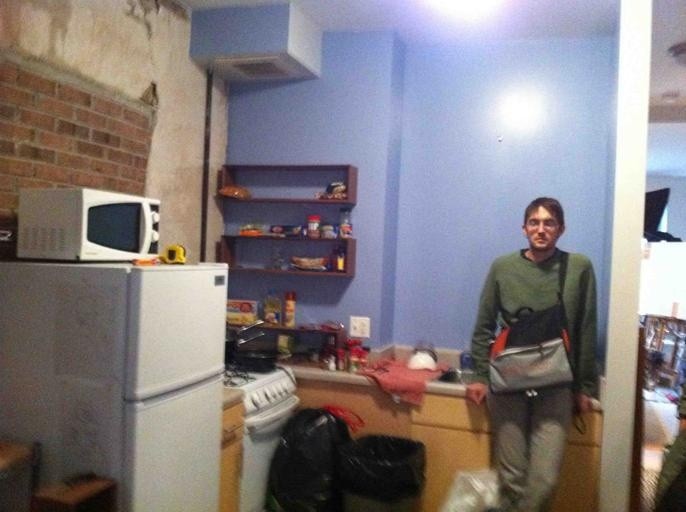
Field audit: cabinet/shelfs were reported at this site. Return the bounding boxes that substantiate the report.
[215,358,605,512]
[217,229,355,279]
[216,161,357,206]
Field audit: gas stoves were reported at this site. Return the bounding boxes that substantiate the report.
[223,363,296,410]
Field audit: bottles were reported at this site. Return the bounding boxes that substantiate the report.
[328,339,371,373]
[282,291,295,328]
[331,249,345,272]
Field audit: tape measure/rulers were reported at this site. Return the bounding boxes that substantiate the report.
[160,244,186,264]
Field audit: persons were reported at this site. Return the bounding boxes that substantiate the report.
[651,364,686,512]
[466,198,599,512]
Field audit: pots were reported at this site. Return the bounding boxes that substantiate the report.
[227,340,277,372]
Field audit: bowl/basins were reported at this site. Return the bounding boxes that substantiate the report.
[272,225,302,236]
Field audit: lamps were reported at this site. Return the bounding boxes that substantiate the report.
[667,42,686,69]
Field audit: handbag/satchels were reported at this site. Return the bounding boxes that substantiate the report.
[489,304,575,395]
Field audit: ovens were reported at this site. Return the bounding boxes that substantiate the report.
[237,395,299,510]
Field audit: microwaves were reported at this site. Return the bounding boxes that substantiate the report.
[15,184,160,262]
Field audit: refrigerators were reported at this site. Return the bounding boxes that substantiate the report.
[0,262,231,512]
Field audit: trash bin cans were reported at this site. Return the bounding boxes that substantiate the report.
[339,435,425,512]
